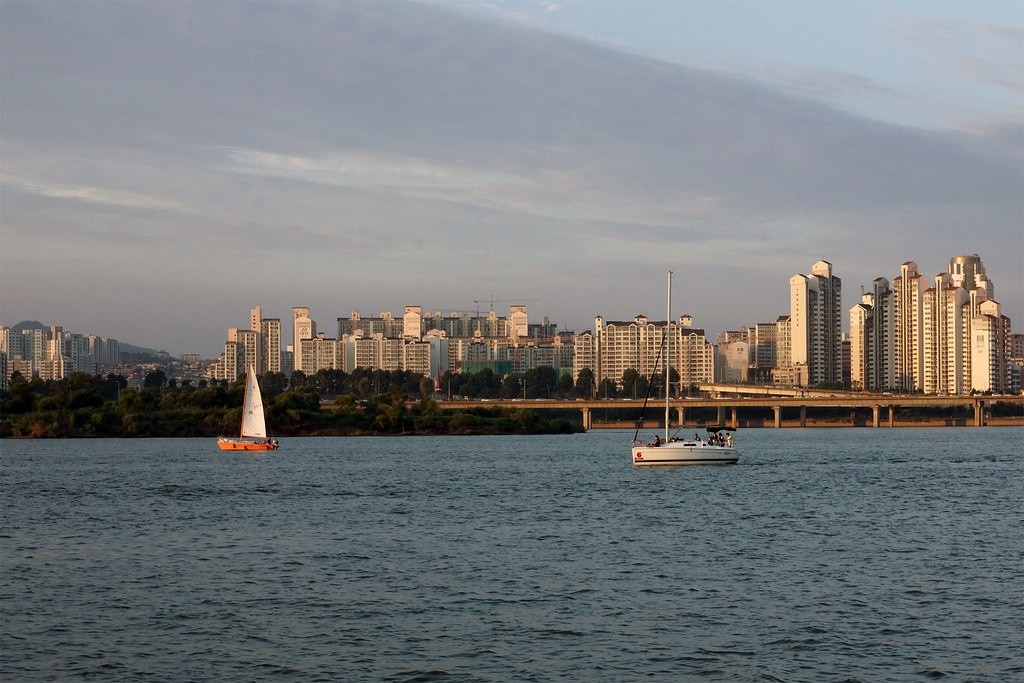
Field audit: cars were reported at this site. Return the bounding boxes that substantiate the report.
[771,395,812,398]
[576,398,586,401]
[763,384,802,389]
[511,399,522,401]
[623,398,633,400]
[882,392,892,395]
[603,398,614,401]
[536,398,544,401]
[937,392,1014,396]
[498,398,504,401]
[646,398,655,400]
[744,397,752,399]
[678,396,733,400]
[481,399,490,402]
[665,397,674,400]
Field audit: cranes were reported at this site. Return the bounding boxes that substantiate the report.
[472,294,542,311]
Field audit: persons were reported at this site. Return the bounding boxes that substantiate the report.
[652,434,660,447]
[694,433,701,442]
[263,437,279,444]
[708,432,735,448]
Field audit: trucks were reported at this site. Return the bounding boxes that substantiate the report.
[453,394,469,401]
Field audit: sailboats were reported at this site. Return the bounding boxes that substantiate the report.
[630,270,740,466]
[217,364,279,451]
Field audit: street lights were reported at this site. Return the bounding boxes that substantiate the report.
[547,384,549,394]
